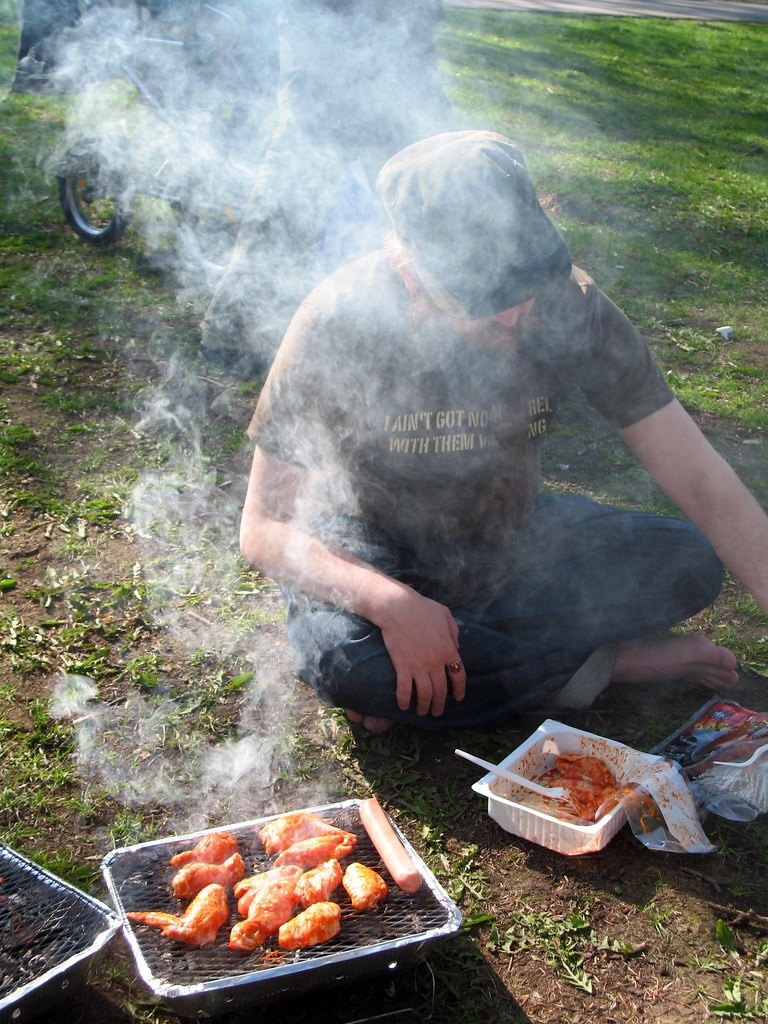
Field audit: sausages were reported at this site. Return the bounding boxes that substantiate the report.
[358,797,422,893]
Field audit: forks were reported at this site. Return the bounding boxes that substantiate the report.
[454,748,585,817]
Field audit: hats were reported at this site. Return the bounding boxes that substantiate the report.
[378,129,572,320]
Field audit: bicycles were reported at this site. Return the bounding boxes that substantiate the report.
[55,0,332,250]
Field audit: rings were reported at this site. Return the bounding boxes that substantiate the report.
[445,659,462,672]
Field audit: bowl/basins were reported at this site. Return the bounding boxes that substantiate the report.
[471,719,665,857]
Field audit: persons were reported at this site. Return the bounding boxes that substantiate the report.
[239,129,767,735]
[197,4,465,365]
